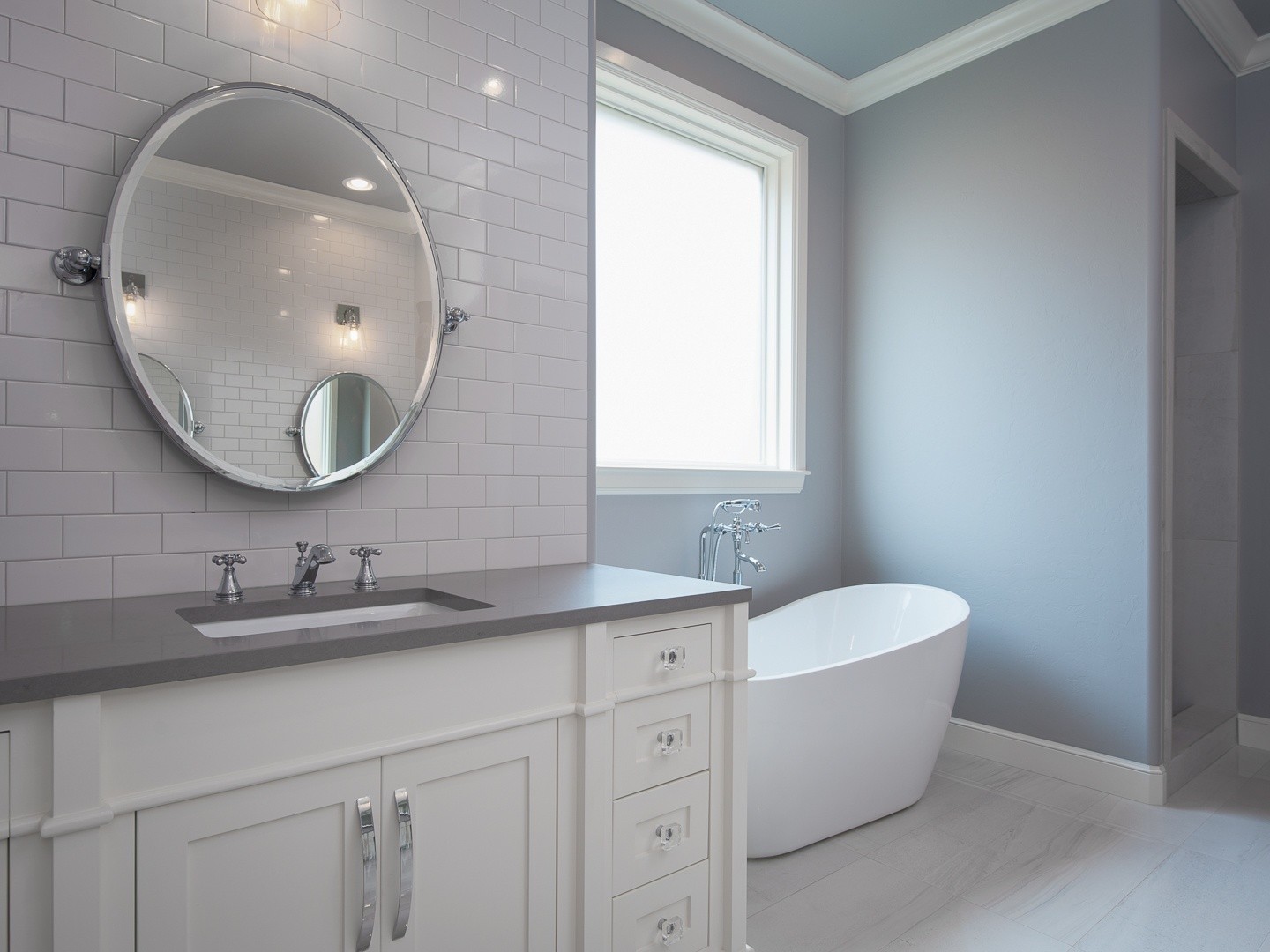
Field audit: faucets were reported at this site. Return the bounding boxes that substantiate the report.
[732,512,767,586]
[288,540,336,598]
[723,498,762,513]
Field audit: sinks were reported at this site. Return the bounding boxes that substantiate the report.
[174,587,496,640]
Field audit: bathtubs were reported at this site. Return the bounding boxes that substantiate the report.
[746,582,971,859]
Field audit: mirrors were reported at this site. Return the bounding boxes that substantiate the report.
[137,352,194,438]
[101,81,445,491]
[299,372,400,479]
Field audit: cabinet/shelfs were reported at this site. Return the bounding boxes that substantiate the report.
[0,603,744,952]
